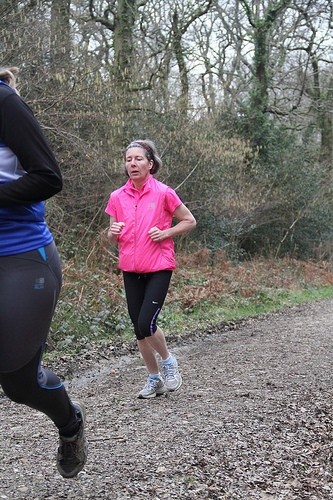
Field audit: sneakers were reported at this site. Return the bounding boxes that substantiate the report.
[55,399,88,478]
[137,374,166,400]
[160,353,183,392]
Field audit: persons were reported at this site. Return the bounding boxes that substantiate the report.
[0,65,88,478]
[105,140,198,399]
